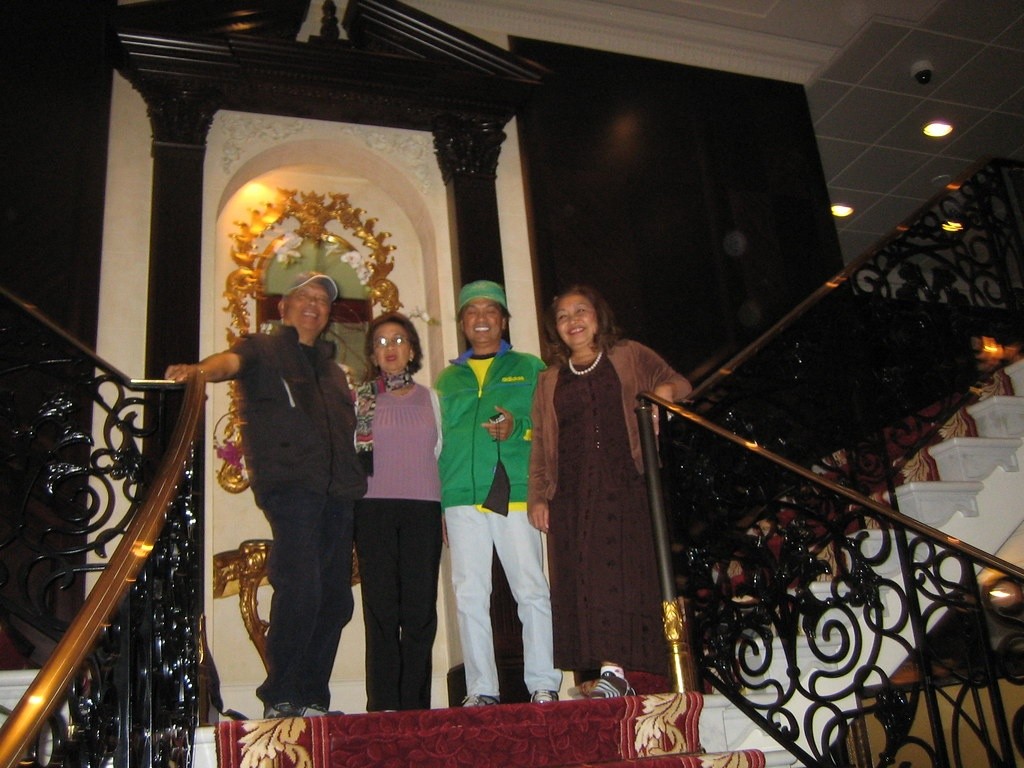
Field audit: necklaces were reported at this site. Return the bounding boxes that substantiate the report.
[567,353,602,375]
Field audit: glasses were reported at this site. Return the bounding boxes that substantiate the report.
[372,336,409,347]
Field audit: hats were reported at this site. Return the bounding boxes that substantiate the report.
[456,280,507,315]
[284,271,338,303]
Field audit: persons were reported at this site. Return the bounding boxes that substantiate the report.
[165,271,368,718]
[526,283,692,699]
[349,312,440,712]
[432,279,563,708]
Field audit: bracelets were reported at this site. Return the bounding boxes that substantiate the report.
[195,364,206,375]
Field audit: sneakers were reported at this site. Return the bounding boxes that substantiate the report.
[263,698,328,717]
[590,671,636,698]
[305,703,344,715]
[530,689,558,703]
[461,693,499,707]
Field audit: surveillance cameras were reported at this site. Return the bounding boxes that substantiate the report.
[911,59,935,84]
